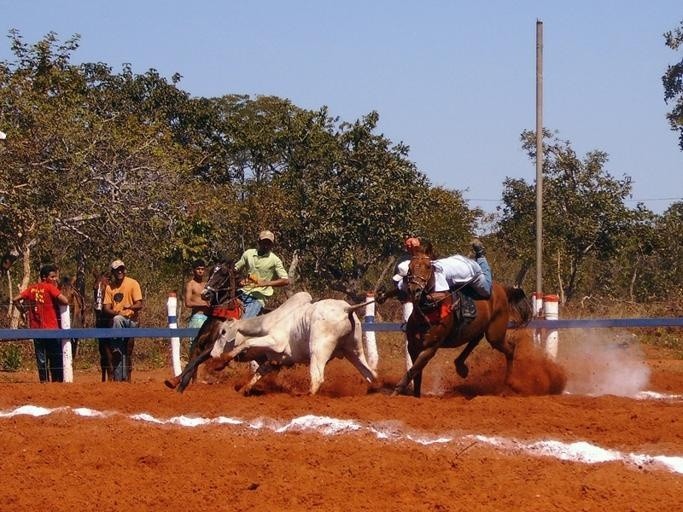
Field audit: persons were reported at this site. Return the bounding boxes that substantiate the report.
[372,238,494,309]
[12,265,70,383]
[231,228,289,320]
[184,258,211,353]
[103,258,143,380]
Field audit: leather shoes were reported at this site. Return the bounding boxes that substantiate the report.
[472,239,485,257]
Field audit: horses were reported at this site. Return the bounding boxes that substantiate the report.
[391,241,534,397]
[92,268,140,384]
[164,254,274,394]
[55,275,87,359]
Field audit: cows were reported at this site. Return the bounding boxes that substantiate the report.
[210,288,409,397]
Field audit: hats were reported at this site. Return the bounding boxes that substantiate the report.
[258,230,274,244]
[393,259,411,282]
[110,259,127,270]
